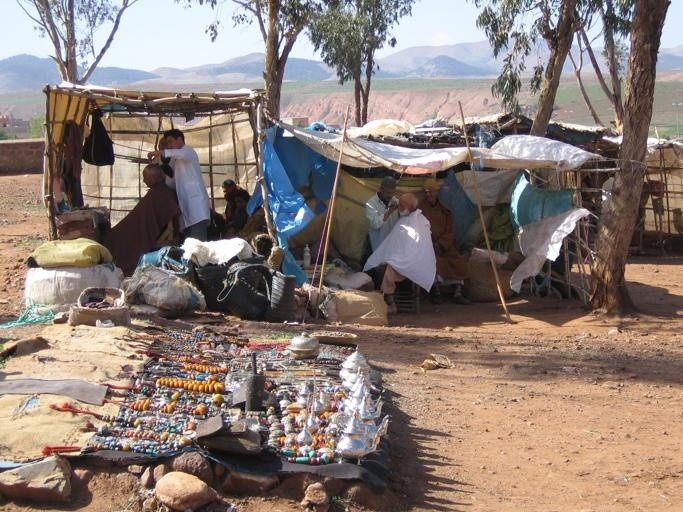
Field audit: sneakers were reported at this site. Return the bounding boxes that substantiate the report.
[450,294,469,305]
[432,292,443,304]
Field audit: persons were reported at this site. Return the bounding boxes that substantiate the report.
[364,175,400,256]
[156,136,167,151]
[415,178,473,306]
[141,163,165,190]
[380,192,419,314]
[221,178,252,233]
[147,128,211,242]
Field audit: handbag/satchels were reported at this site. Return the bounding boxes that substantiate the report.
[196,416,261,454]
[142,246,296,322]
[84,111,114,165]
